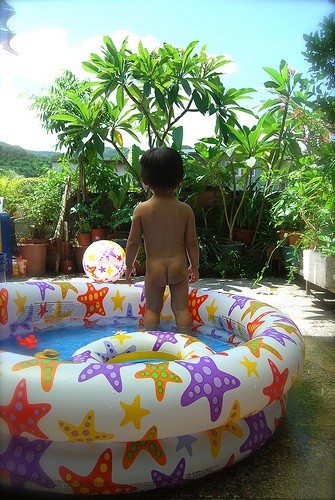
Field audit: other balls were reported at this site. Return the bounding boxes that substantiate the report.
[83,239,127,284]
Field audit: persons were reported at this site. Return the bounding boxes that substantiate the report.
[125,147,200,329]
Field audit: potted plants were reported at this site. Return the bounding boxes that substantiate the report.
[15,38,335,295]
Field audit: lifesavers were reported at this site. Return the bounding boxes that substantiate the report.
[69,330,218,363]
